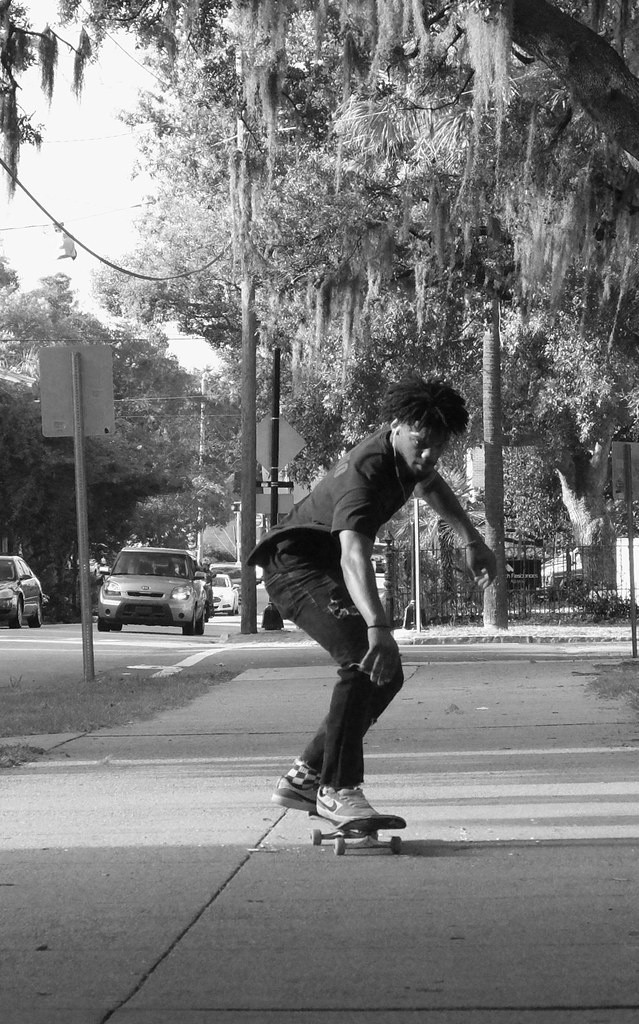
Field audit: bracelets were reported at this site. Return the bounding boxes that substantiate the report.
[466,533,487,549]
[364,625,393,633]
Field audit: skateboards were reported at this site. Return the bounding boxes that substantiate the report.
[308,801,407,856]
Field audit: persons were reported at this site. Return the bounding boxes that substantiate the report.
[246,372,497,825]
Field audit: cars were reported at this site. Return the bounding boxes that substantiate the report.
[211,574,241,616]
[185,549,262,623]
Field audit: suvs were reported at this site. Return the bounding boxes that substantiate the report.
[0,555,44,629]
[97,546,207,636]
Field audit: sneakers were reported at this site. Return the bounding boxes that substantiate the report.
[271,756,321,819]
[318,785,380,822]
